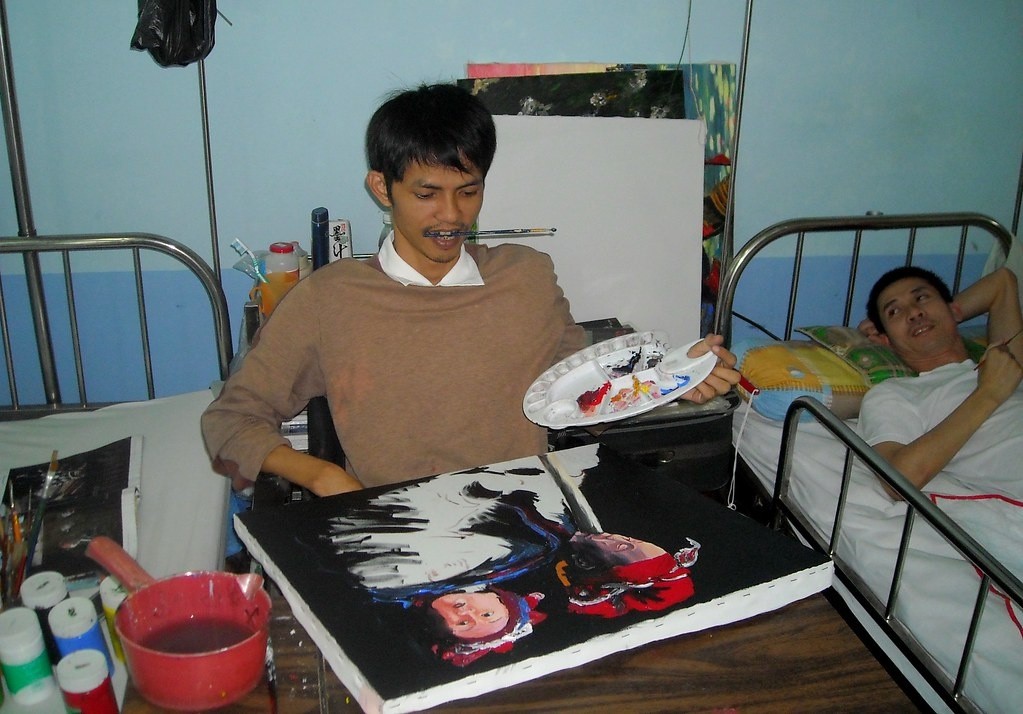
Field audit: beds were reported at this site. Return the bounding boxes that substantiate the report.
[0,234,237,714]
[713,212,1023,714]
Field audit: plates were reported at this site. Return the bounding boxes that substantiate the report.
[522,331,718,429]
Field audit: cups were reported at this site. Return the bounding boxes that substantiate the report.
[250,273,299,318]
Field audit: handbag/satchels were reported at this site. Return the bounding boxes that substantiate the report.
[130,0,231,68]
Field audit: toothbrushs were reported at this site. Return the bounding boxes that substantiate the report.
[229,238,269,283]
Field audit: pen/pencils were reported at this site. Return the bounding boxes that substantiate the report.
[10,509,24,543]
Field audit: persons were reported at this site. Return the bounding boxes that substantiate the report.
[857,266,1023,500]
[201,83,742,499]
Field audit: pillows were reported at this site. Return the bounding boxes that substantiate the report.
[793,324,988,387]
[729,342,873,424]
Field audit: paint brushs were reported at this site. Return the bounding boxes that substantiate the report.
[265,636,279,713]
[0,450,60,607]
[424,226,557,237]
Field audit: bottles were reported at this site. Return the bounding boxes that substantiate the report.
[0,571,129,714]
[379,213,394,248]
[266,242,299,272]
